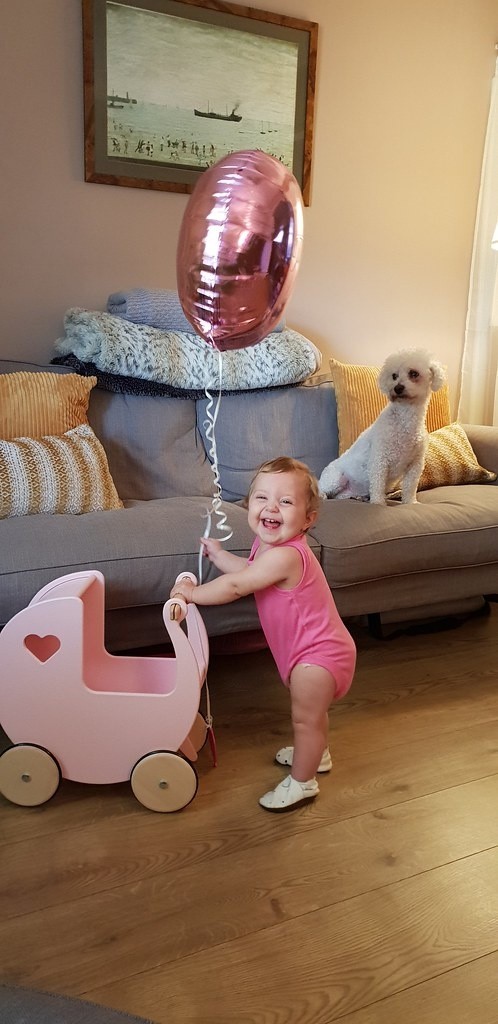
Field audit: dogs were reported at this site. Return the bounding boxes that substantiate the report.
[317,348,445,506]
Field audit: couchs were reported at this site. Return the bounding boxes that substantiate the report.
[0,355,498,652]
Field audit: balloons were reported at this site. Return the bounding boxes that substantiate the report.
[176,149,303,353]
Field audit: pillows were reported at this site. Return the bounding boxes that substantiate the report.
[86,387,220,497]
[1,425,126,521]
[192,382,339,501]
[330,357,451,457]
[0,370,98,441]
[384,421,498,498]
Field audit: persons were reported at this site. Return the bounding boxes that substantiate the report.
[111,120,283,169]
[169,456,357,814]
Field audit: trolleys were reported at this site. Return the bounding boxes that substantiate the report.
[1,568,210,813]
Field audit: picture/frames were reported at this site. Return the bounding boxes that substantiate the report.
[82,1,319,207]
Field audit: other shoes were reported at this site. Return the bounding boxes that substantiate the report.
[259,774,320,814]
[276,746,333,772]
